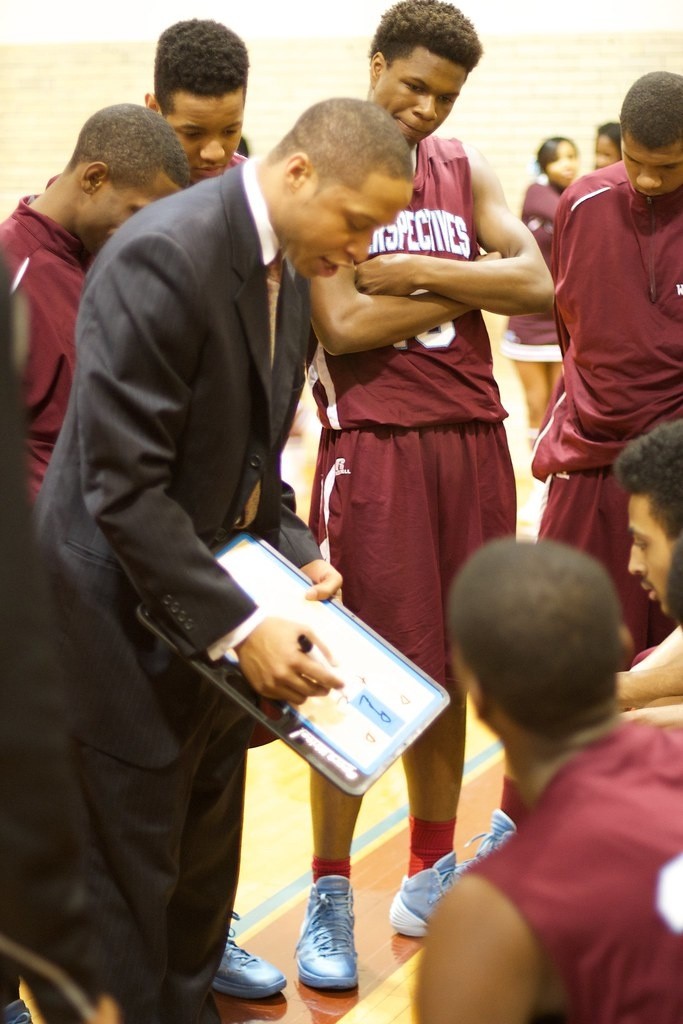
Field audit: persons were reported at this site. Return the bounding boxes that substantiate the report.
[33,97,414,1023]
[454,69,682,883]
[294,1,556,991]
[594,122,623,174]
[414,539,683,1023]
[611,422,683,714]
[144,19,255,187]
[499,137,580,450]
[0,103,194,1023]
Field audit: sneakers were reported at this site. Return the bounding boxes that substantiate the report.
[293,875,358,988]
[388,851,460,936]
[454,809,517,878]
[211,911,287,997]
[1,998,33,1024]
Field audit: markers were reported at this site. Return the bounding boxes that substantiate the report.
[298,634,336,676]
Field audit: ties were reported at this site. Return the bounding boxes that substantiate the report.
[233,248,283,529]
